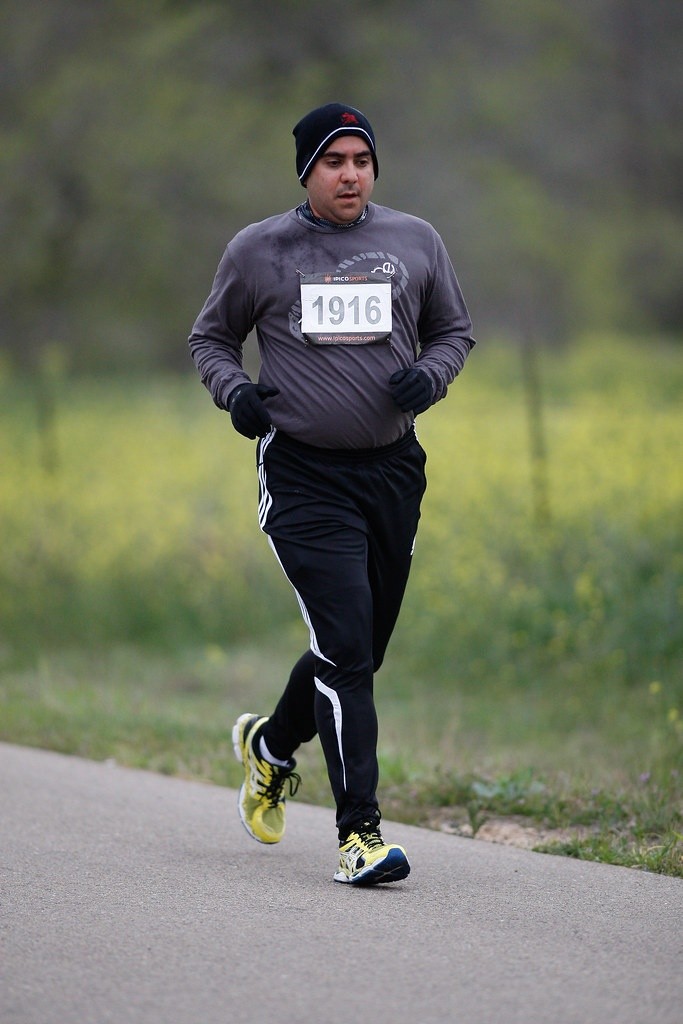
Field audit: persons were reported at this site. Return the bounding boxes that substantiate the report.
[188,104,476,884]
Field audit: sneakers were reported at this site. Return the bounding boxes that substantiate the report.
[231,713,301,842]
[334,824,411,884]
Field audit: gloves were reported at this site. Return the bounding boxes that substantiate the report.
[226,384,282,440]
[390,369,434,415]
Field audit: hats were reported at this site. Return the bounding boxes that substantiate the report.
[292,103,380,188]
